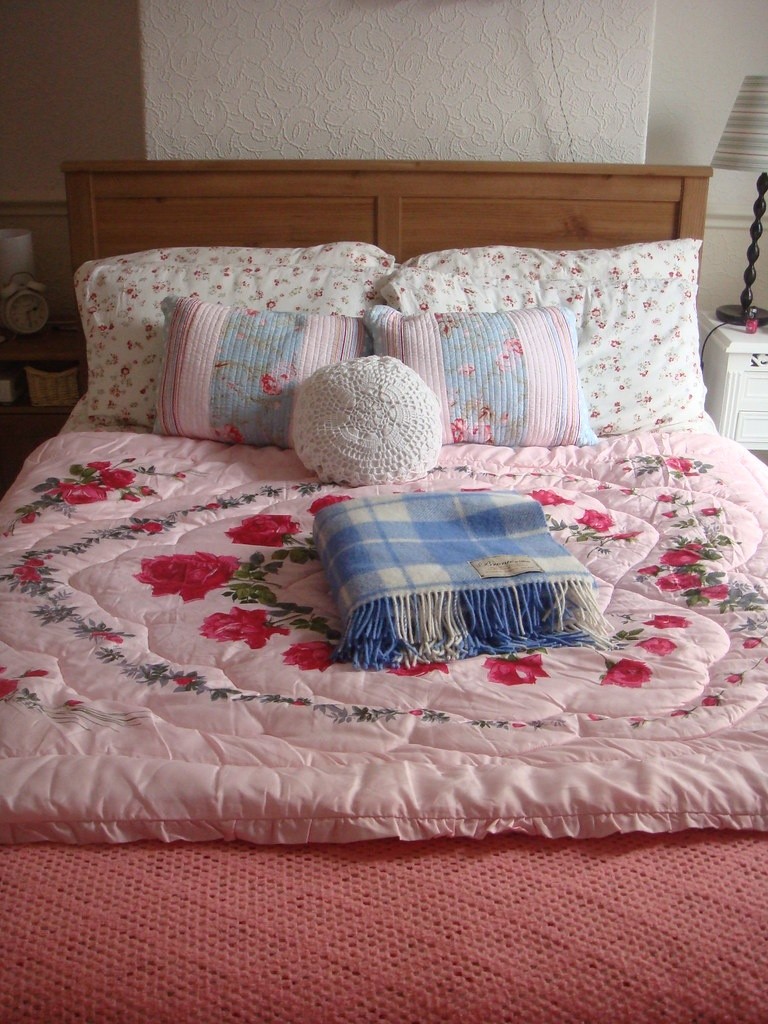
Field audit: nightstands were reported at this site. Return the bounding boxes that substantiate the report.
[0,315,88,500]
[696,309,768,451]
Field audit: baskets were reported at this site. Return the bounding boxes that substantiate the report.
[26,365,80,407]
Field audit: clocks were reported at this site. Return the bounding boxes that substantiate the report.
[0,272,49,339]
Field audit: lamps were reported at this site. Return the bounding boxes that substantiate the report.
[710,76,768,325]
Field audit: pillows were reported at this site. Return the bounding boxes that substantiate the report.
[289,355,445,486]
[73,241,395,428]
[382,239,709,436]
[363,305,600,448]
[154,295,372,450]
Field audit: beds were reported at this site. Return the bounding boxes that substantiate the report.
[0,159,768,1024]
